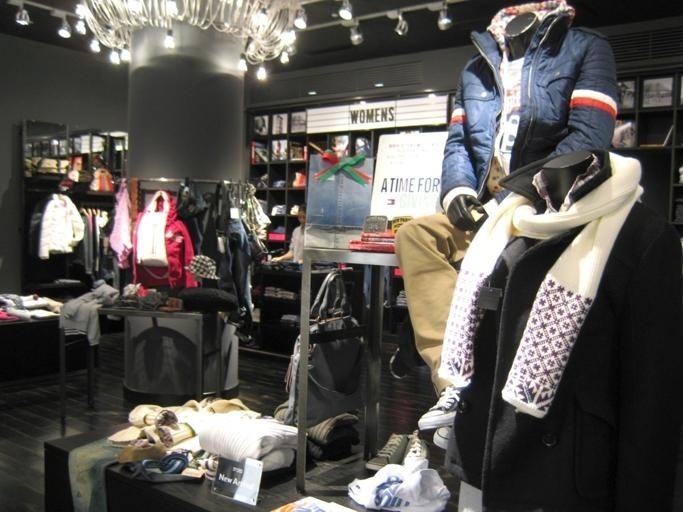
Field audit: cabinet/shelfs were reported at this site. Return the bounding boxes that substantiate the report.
[0,62,683,511]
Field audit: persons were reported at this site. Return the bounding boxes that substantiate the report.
[452,152,683,508]
[394,0,620,450]
[134,189,194,287]
[37,194,84,260]
[273,205,306,263]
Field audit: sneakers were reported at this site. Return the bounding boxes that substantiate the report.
[418,385,464,431]
[365,432,430,471]
[432,427,451,449]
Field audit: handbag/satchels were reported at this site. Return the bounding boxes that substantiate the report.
[137,211,169,268]
[90,168,114,192]
[237,193,271,260]
[284,317,363,428]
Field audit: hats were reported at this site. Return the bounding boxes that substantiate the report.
[185,256,222,280]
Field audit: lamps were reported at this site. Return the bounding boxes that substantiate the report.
[14,0,452,82]
